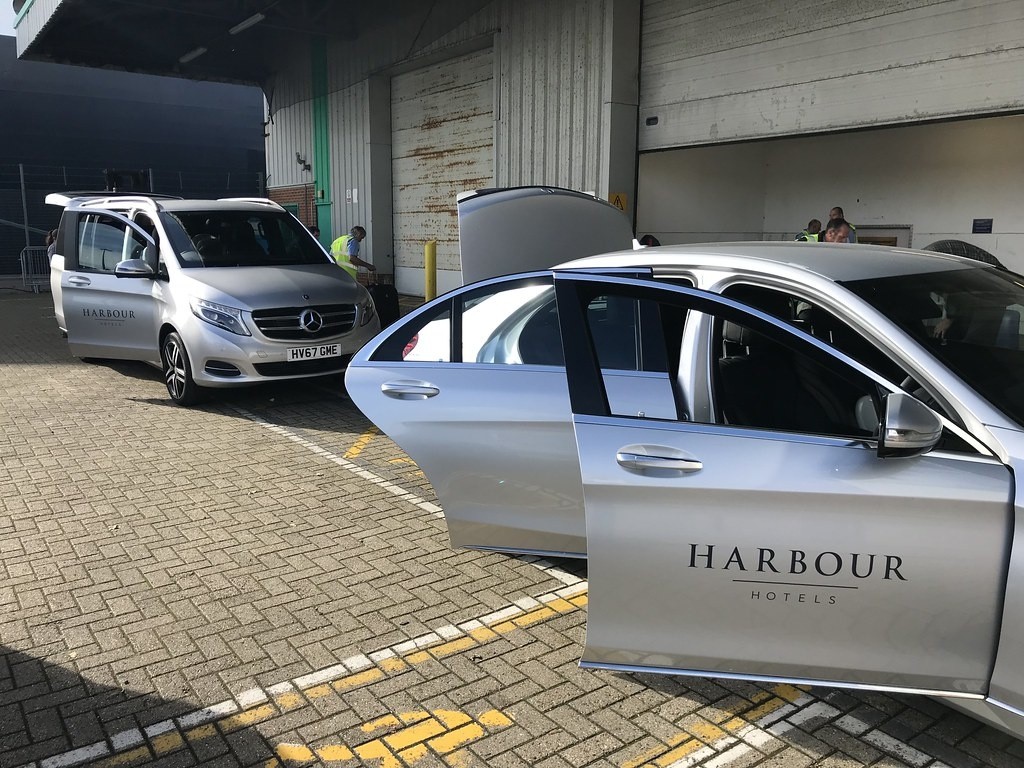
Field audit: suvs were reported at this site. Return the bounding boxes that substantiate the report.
[43,192,384,404]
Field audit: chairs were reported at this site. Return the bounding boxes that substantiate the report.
[713,289,827,437]
[791,296,899,441]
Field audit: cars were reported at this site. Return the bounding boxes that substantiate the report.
[341,186,1023,742]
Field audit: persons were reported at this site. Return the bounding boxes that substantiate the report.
[829,206,859,243]
[307,225,321,242]
[46,228,59,268]
[595,239,1024,460]
[793,217,851,243]
[795,218,822,240]
[328,225,377,284]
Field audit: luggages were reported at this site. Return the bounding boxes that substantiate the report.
[364,266,401,339]
